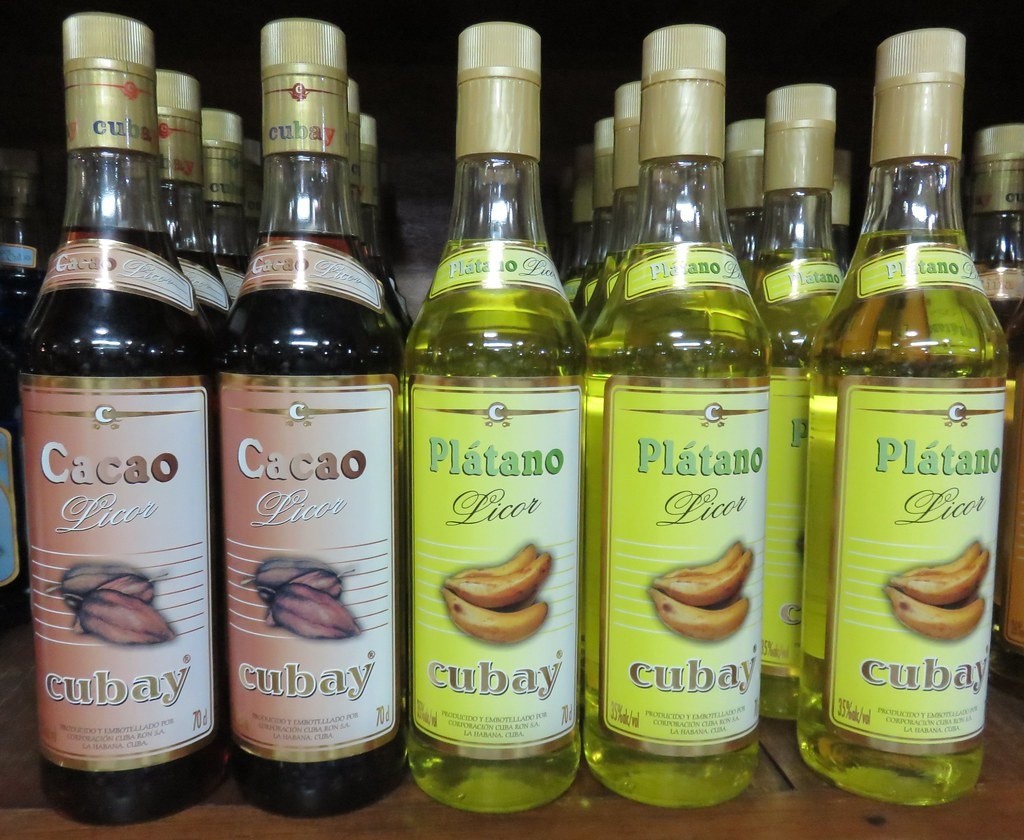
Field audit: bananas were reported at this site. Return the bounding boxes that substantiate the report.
[888,540,992,643]
[646,538,752,643]
[438,541,551,642]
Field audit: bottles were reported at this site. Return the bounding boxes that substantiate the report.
[0,6,1023,817]
